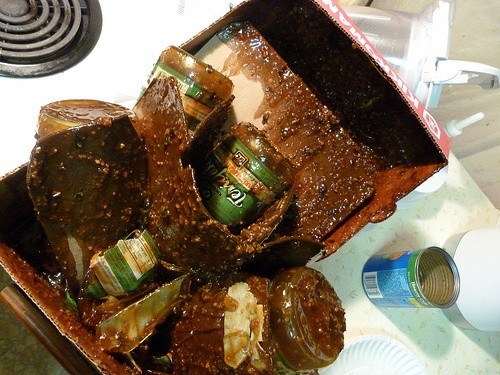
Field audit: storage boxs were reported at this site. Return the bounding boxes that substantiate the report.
[0,0,453,375]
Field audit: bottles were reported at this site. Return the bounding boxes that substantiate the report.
[198,121,292,231]
[263,268,346,373]
[29,100,135,301]
[136,47,234,145]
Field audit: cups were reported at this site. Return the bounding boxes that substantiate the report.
[361,247,459,309]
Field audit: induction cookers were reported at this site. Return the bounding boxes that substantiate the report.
[0,0,103,79]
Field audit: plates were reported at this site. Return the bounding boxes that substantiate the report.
[454,229,500,332]
